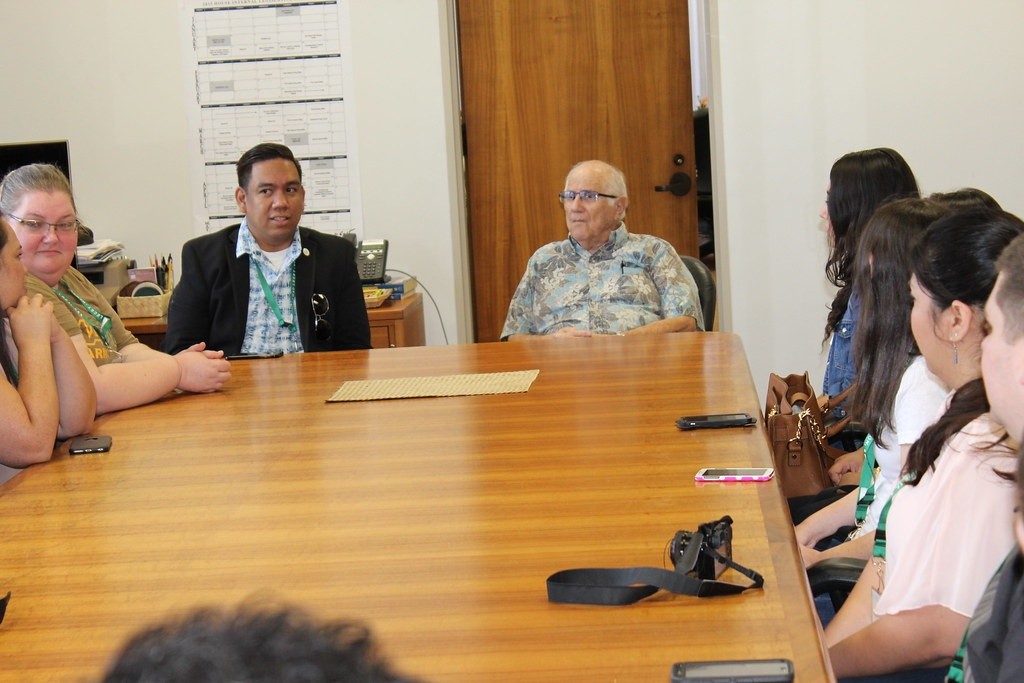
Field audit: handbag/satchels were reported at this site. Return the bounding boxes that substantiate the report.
[762,371,869,498]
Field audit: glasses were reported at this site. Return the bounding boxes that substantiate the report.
[5,210,80,234]
[559,190,621,204]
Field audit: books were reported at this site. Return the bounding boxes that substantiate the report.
[76,240,123,264]
[376,276,418,301]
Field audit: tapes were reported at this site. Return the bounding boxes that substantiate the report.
[131,281,164,297]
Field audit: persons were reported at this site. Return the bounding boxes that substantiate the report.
[787,186,1001,526]
[107,600,397,683]
[794,197,951,628]
[944,230,1024,683]
[0,164,232,416]
[500,160,705,343]
[817,148,921,452]
[824,209,1024,683]
[164,143,372,359]
[0,218,97,486]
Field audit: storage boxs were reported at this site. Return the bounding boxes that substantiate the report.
[116,292,172,318]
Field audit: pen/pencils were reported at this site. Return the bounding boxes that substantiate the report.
[161,253,173,289]
[155,254,158,268]
[149,256,153,267]
[621,260,625,274]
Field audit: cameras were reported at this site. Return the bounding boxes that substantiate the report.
[670,517,734,579]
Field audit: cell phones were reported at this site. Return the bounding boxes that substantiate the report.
[68,436,112,455]
[679,413,753,428]
[670,659,795,683]
[694,468,775,482]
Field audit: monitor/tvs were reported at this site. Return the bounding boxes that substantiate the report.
[0,140,72,192]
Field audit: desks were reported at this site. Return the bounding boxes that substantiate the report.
[122,291,426,351]
[0,332,837,683]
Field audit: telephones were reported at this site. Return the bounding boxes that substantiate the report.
[342,233,389,284]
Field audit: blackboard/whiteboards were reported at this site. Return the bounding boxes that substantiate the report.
[0,139,78,270]
